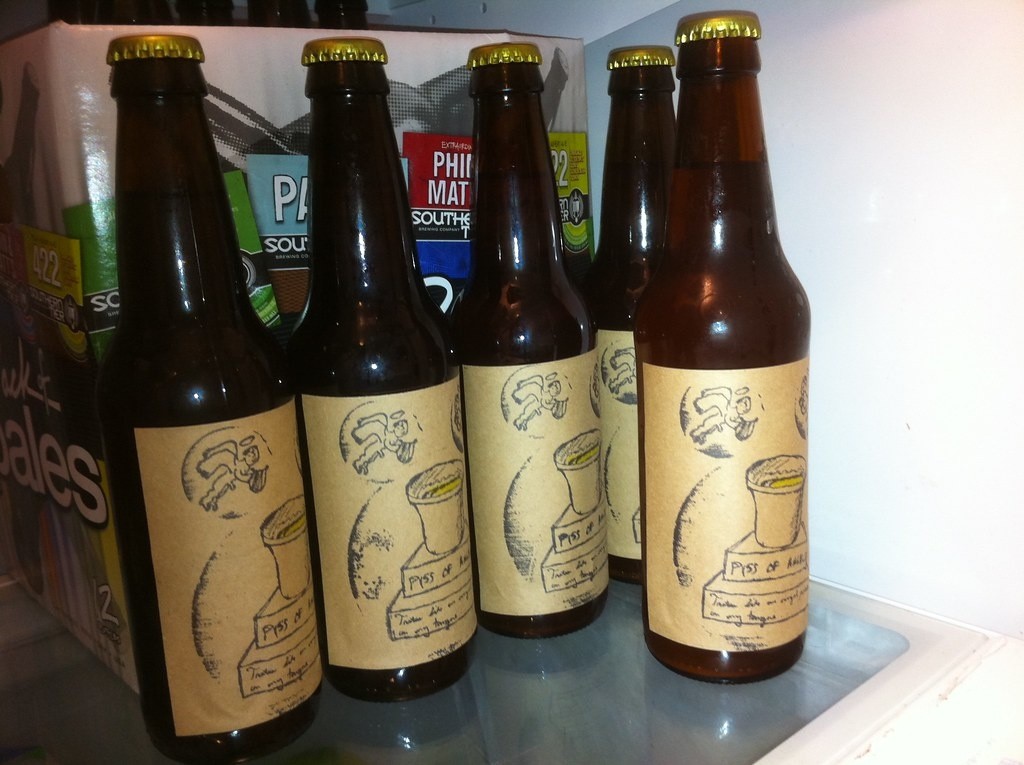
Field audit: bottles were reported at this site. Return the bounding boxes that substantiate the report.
[283,36,480,703]
[636,7,812,687]
[95,32,327,765]
[451,43,615,641]
[595,45,682,586]
